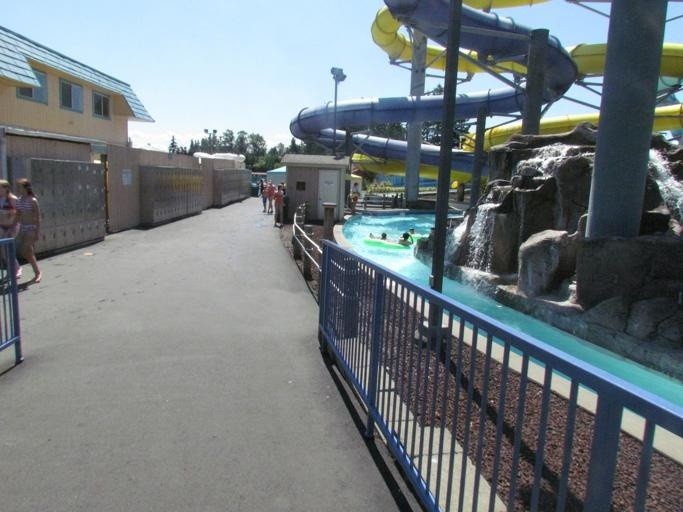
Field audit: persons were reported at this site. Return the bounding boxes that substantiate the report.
[409,228,414,234]
[13,179,42,283]
[387,232,413,245]
[350,182,360,213]
[369,232,387,240]
[391,192,403,207]
[0,180,22,279]
[258,179,287,226]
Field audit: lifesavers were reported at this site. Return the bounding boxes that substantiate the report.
[365,239,410,248]
[409,234,424,241]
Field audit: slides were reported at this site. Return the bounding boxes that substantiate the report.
[349,0,683,188]
[289,0,576,176]
